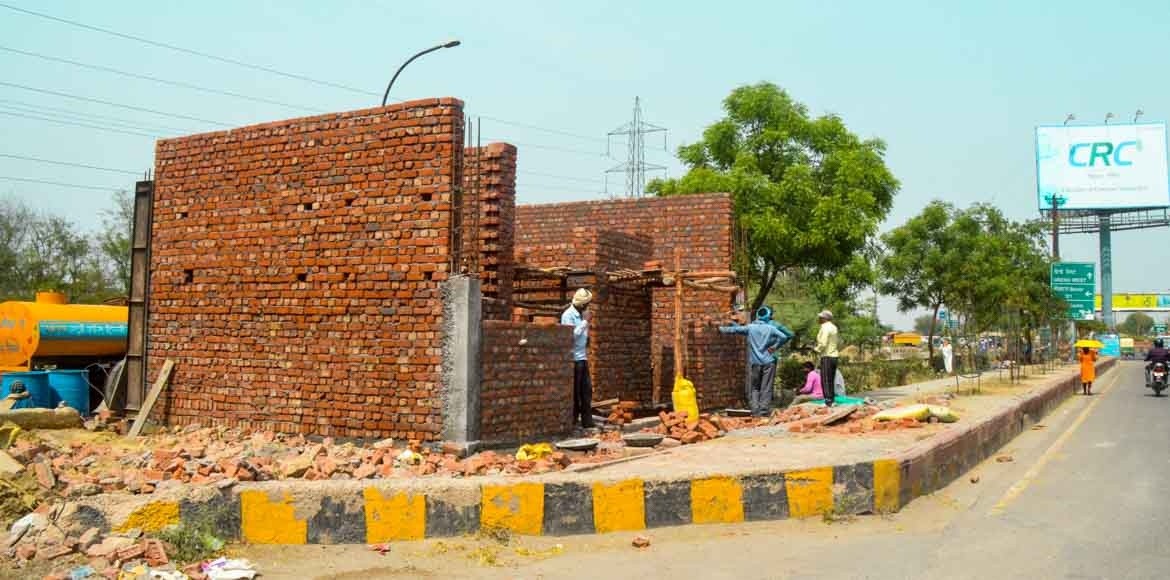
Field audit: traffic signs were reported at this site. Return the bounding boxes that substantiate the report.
[1047,262,1096,321]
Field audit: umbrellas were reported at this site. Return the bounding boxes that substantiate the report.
[1072,339,1104,348]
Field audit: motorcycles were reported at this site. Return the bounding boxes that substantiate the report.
[1143,359,1170,395]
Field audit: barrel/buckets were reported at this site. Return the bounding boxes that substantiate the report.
[0,371,51,409]
[46,369,89,417]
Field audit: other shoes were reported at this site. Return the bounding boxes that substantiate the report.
[1146,384,1151,387]
[583,423,602,430]
[817,404,833,409]
[1164,384,1168,387]
[760,409,769,413]
[752,411,760,416]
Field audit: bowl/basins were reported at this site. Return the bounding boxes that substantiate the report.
[556,439,599,450]
[624,434,664,447]
[726,409,751,417]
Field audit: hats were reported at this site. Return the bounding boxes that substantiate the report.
[818,310,833,319]
[572,288,592,304]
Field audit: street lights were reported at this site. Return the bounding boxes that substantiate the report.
[381,39,461,107]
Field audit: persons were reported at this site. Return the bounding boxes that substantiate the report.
[939,338,953,373]
[833,366,846,396]
[561,288,600,431]
[814,310,839,408]
[1079,347,1097,395]
[1144,338,1170,387]
[726,305,795,399]
[787,361,824,408]
[717,307,787,416]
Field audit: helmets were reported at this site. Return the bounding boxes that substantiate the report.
[1154,338,1163,346]
